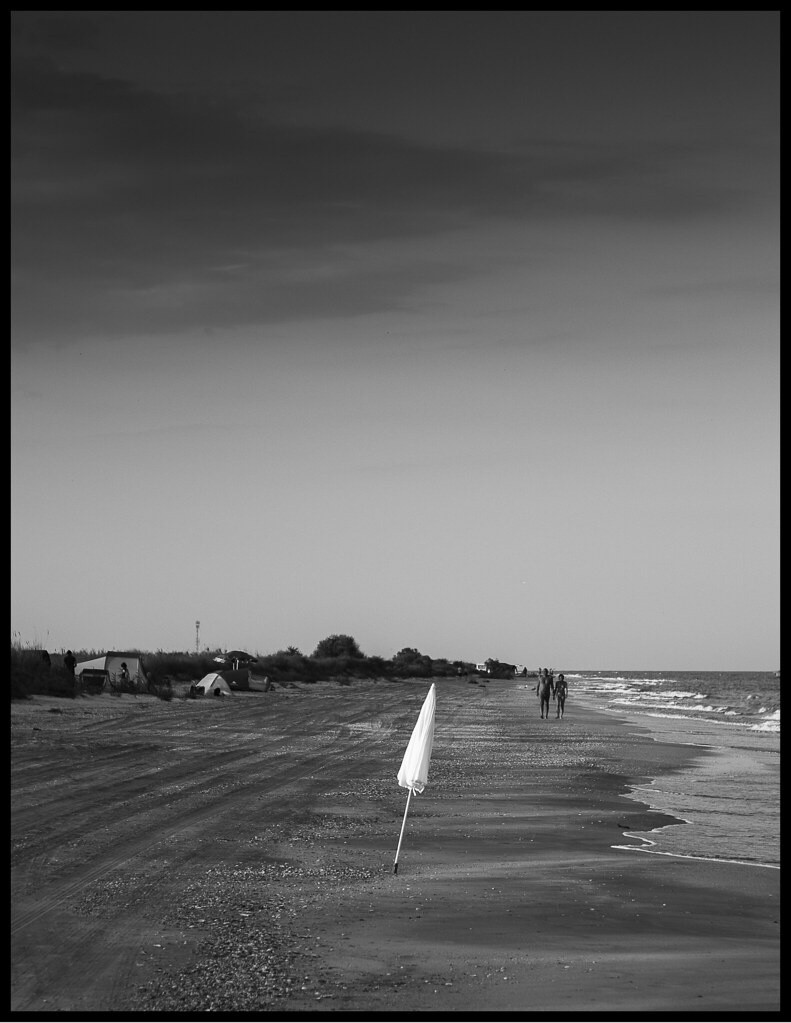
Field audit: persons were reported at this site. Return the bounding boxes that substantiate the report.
[552,673,568,720]
[536,667,555,719]
[64,650,78,677]
[119,662,131,688]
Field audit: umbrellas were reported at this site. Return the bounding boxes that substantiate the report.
[381,682,438,876]
[217,650,257,670]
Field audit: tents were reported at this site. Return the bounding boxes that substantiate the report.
[216,668,270,692]
[195,673,233,697]
[74,651,152,692]
